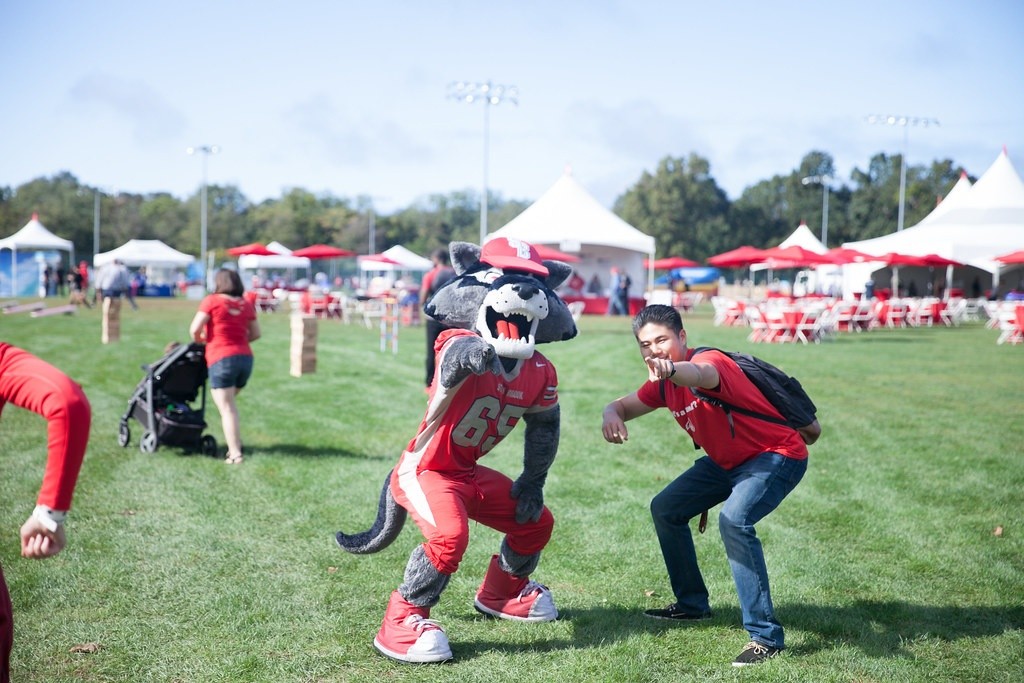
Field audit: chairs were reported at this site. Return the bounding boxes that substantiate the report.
[711,297,1024,345]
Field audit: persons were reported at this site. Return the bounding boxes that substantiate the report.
[420,248,458,389]
[189,270,263,463]
[39,257,146,311]
[0,341,92,683]
[601,302,822,668]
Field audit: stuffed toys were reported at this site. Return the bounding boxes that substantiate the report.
[336,237,578,662]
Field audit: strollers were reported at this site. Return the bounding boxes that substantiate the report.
[117,342,219,458]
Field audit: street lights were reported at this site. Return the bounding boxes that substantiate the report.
[801,175,842,249]
[187,145,221,286]
[446,80,517,245]
[865,114,940,297]
[77,186,118,255]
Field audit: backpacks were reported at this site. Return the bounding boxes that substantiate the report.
[660,346,821,445]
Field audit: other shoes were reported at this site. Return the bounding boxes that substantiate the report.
[225,454,243,464]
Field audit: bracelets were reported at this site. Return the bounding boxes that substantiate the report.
[668,360,675,381]
[31,502,66,533]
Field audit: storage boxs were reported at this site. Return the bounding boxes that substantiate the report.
[289,315,317,378]
[101,296,120,344]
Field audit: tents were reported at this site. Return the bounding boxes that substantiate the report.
[0,213,76,299]
[92,238,196,300]
[646,146,1022,310]
[483,176,658,320]
[227,241,434,299]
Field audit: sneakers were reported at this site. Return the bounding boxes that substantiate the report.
[643,604,712,621]
[731,641,780,665]
